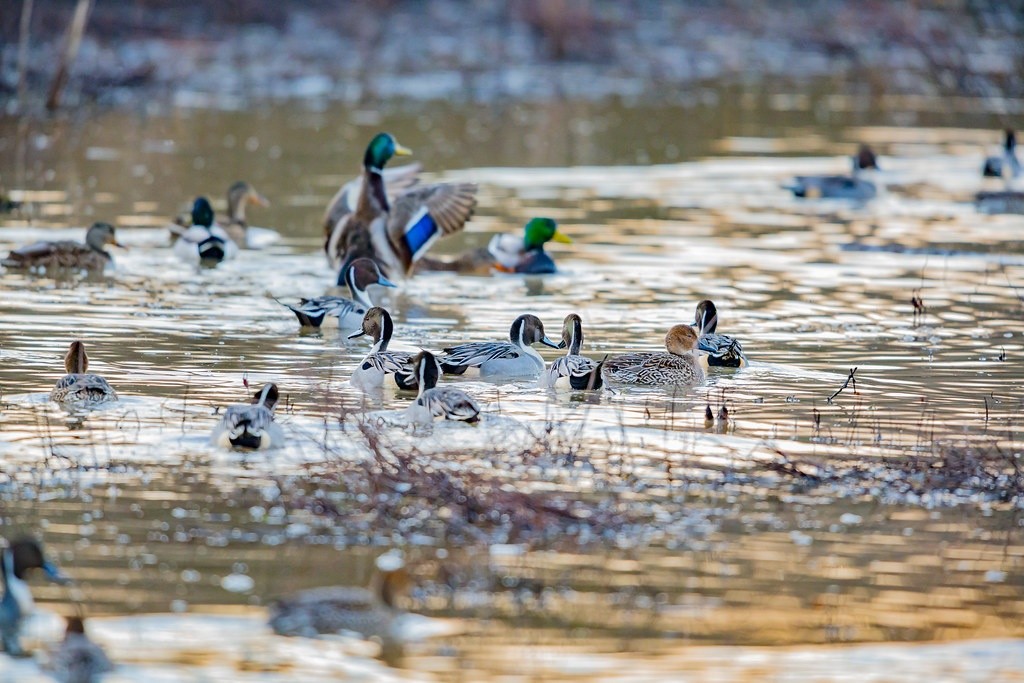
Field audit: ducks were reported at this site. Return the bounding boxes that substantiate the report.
[49,341,118,404]
[271,258,398,329]
[417,217,575,277]
[0,222,126,275]
[1,530,116,683]
[266,551,484,644]
[208,382,285,453]
[433,314,559,378]
[606,324,706,386]
[778,143,881,199]
[547,313,608,391]
[169,181,270,249]
[690,300,749,367]
[322,133,478,286]
[349,306,443,395]
[982,127,1024,178]
[410,350,482,424]
[174,197,239,268]
